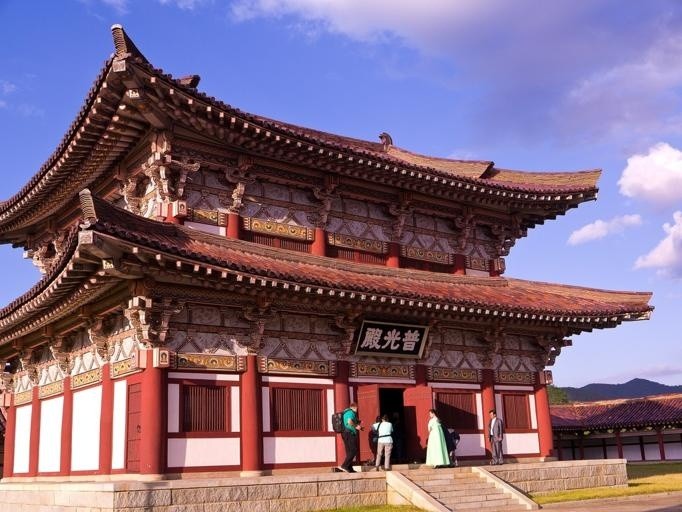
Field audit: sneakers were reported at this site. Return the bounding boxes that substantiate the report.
[339,464,350,473]
[349,465,357,473]
[385,468,391,471]
[376,466,379,471]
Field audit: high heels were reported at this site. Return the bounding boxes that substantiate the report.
[432,465,440,469]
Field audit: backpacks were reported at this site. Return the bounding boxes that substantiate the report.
[332,409,351,432]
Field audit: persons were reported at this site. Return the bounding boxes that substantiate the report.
[373,415,395,472]
[389,411,408,464]
[425,409,450,469]
[340,402,364,473]
[487,410,505,464]
[368,416,381,462]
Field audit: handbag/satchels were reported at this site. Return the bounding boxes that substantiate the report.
[371,422,382,443]
